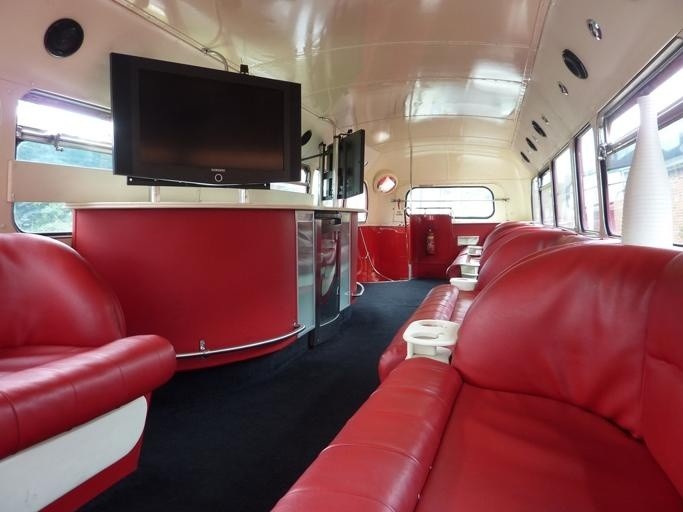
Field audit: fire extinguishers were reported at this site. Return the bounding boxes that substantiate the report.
[426,224,435,255]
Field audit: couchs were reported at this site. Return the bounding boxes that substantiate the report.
[377,219,591,386]
[1,229,176,510]
[268,242,683,510]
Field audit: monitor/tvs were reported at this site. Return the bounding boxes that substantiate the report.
[109,51,301,189]
[325,129,365,199]
